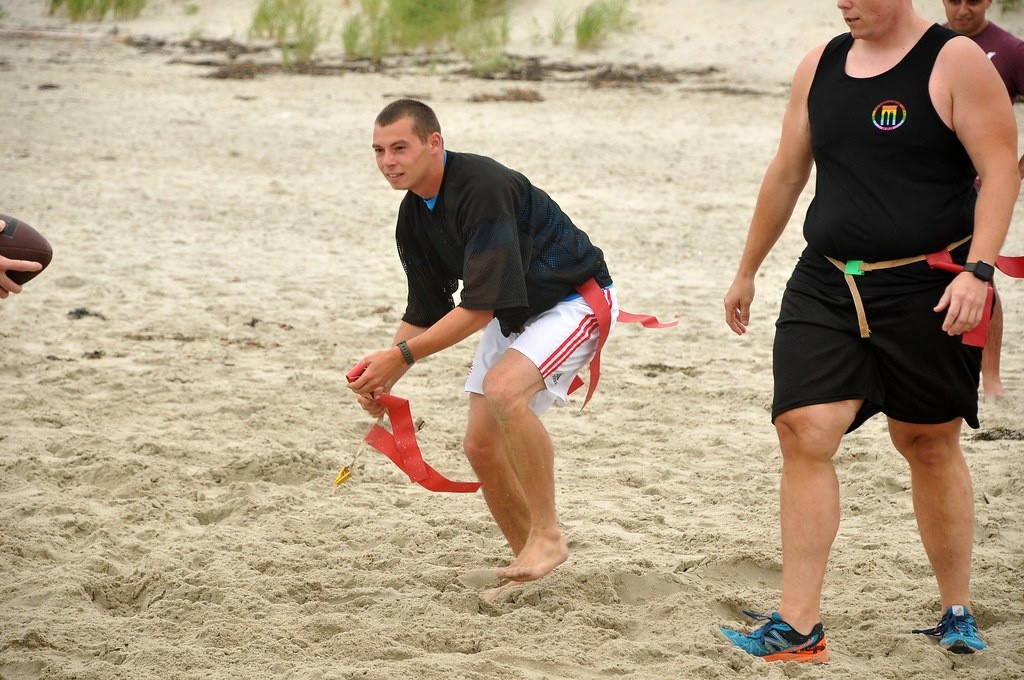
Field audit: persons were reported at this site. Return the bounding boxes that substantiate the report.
[941,0,1024,404]
[720,0,1021,664]
[346,99,619,587]
[0,220,42,299]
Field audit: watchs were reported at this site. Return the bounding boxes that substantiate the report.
[962,260,994,282]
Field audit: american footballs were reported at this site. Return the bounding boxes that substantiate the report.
[0,213,53,286]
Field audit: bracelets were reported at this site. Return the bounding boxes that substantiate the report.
[396,340,414,365]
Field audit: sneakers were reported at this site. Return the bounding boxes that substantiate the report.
[912,605,991,654]
[720,610,829,664]
